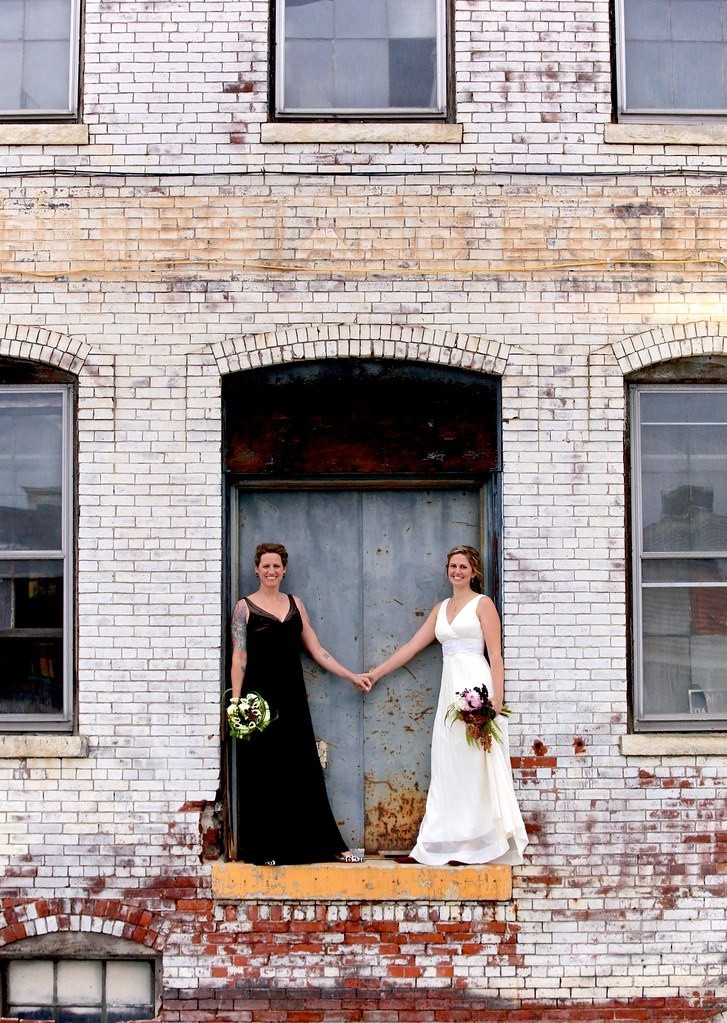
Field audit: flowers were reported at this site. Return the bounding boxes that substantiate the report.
[445,684,509,753]
[226,692,271,740]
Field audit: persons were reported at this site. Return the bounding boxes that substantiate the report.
[229,542,373,865]
[355,545,529,867]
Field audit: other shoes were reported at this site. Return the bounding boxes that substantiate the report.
[266,860,276,866]
[336,854,364,863]
[393,857,418,863]
[448,861,460,866]
